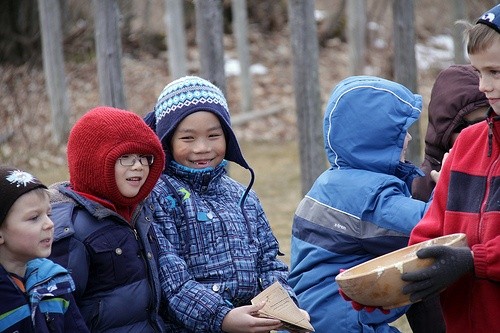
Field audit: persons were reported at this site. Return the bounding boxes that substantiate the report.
[339,4,500,333]
[143,75,310,333]
[46,107,166,333]
[0,165,90,333]
[406,64,492,333]
[289,75,452,333]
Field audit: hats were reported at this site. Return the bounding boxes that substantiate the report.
[476,3,500,33]
[154,75,250,173]
[0,167,48,227]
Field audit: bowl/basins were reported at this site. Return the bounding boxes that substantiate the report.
[334,233,467,311]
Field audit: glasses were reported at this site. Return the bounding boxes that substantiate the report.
[452,118,487,133]
[115,153,155,167]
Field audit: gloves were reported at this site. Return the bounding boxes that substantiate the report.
[334,245,476,315]
